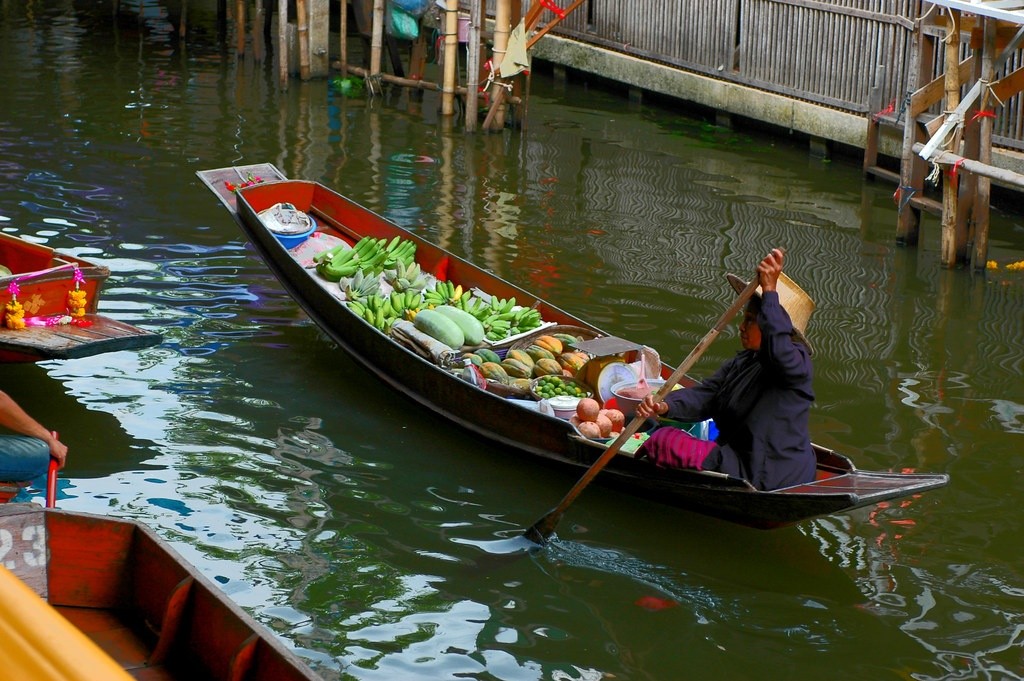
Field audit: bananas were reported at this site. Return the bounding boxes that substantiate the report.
[310,234,543,344]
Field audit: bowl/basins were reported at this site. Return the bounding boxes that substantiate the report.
[593,414,657,444]
[272,215,317,250]
[530,374,594,419]
[612,379,669,412]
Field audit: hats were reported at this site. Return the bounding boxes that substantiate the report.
[726,270,817,356]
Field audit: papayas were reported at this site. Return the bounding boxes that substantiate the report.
[456,332,589,387]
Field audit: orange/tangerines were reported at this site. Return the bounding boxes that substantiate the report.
[534,375,586,399]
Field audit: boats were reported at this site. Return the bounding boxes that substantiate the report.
[0,230,163,365]
[195,163,950,529]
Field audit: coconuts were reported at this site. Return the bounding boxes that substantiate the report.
[570,398,625,439]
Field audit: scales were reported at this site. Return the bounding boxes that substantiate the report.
[568,336,646,406]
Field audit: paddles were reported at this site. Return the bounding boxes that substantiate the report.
[521,244,789,548]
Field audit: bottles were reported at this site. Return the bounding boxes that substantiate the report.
[463,358,479,387]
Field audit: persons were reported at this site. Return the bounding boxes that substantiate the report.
[634,247,819,490]
[0,389,69,481]
